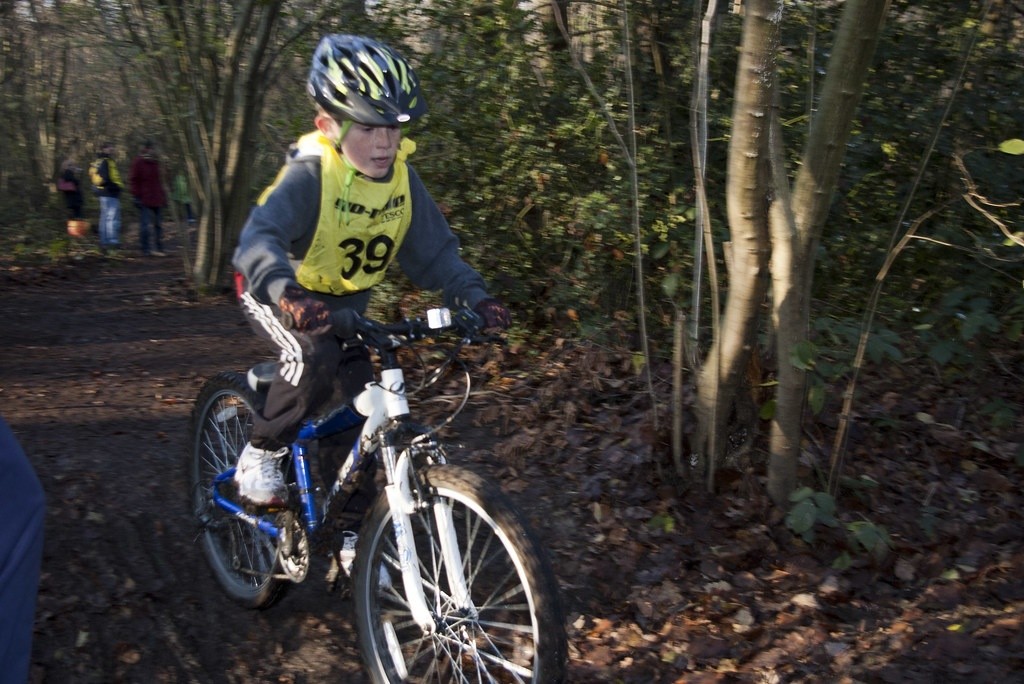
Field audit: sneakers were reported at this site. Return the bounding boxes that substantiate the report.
[339,531,392,589]
[233,440,289,505]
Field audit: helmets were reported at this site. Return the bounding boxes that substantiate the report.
[306,32,428,127]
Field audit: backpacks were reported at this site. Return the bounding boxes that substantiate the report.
[89,158,106,189]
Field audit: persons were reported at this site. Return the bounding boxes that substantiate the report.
[128,142,169,257]
[173,162,199,226]
[231,34,513,592]
[51,158,90,240]
[88,141,126,248]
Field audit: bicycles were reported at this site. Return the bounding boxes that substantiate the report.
[185,304,569,683]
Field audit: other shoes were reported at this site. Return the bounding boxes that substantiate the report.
[153,250,168,256]
